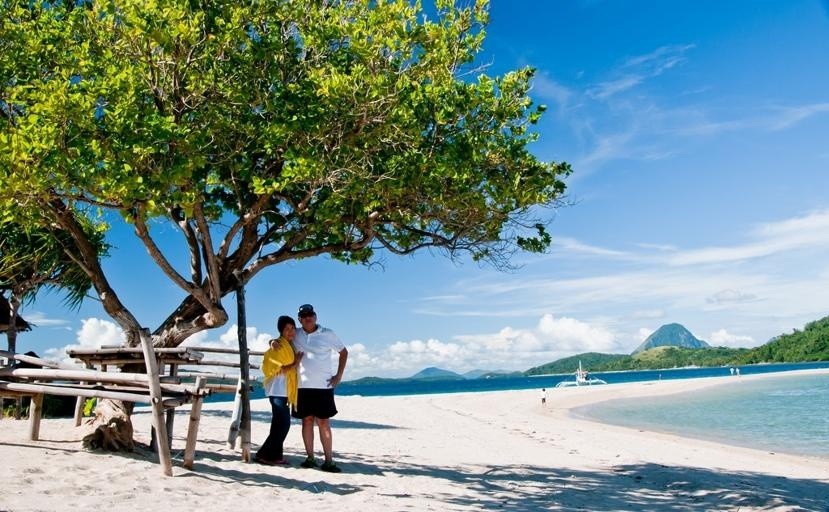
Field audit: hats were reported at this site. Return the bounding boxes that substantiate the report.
[298,304,315,316]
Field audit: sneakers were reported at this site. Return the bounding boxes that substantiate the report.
[301,457,318,468]
[253,452,275,466]
[320,462,341,473]
[271,455,289,464]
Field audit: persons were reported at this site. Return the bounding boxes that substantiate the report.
[269,304,347,473]
[253,315,304,465]
